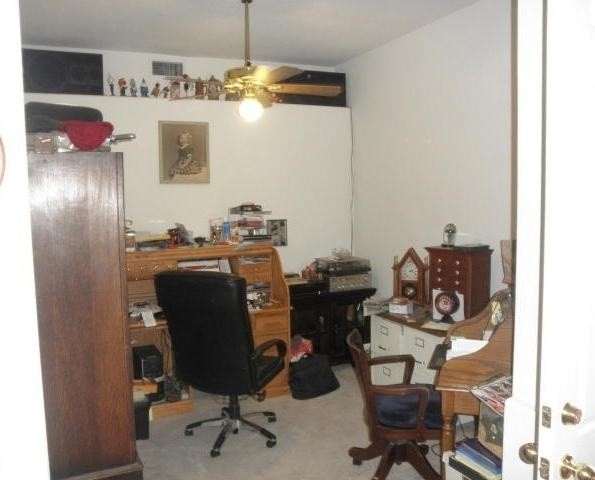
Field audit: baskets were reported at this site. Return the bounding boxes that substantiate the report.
[246,289,271,305]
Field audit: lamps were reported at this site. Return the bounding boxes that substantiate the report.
[238,84,263,120]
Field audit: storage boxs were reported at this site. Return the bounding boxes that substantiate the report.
[133,390,151,440]
[478,402,503,459]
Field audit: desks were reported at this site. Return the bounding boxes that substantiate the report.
[125,243,295,422]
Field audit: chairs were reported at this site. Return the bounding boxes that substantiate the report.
[346,328,441,480]
[154,269,288,457]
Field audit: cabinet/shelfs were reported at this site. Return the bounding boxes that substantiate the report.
[288,280,377,368]
[424,245,494,319]
[371,304,453,387]
[27,151,144,480]
[429,287,513,480]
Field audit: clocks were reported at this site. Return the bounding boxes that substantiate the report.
[391,247,430,305]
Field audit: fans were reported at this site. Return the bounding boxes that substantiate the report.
[162,0,342,101]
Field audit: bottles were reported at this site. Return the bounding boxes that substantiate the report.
[221,217,231,240]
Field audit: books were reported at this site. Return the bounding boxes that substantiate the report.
[230,204,273,244]
[448,375,512,479]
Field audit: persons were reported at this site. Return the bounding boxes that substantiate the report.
[168,130,200,177]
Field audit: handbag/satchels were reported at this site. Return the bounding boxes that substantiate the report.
[288,353,340,399]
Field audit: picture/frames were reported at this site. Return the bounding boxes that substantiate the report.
[158,120,211,184]
[266,219,288,246]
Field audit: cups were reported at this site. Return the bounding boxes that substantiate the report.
[125,231,136,252]
